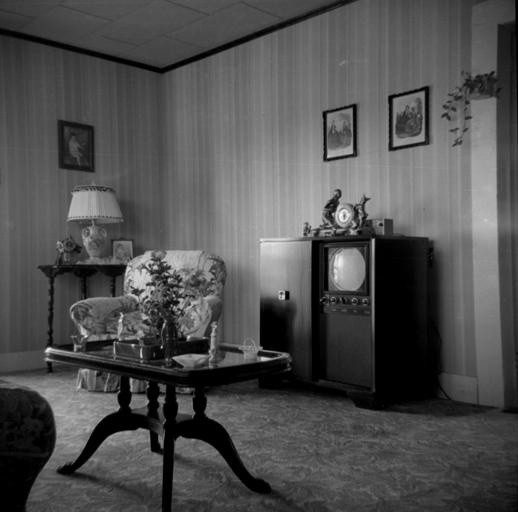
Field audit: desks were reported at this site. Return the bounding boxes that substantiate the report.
[36,264,126,374]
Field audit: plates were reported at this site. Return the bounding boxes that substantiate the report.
[172,353,211,369]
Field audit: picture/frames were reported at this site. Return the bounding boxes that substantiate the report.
[322,104,357,161]
[111,239,134,261]
[388,86,429,151]
[57,119,95,172]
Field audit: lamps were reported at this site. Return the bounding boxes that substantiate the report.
[66,185,124,257]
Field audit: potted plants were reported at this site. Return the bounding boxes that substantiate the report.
[114,248,208,362]
[441,71,504,146]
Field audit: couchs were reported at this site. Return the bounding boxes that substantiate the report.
[0,379,56,512]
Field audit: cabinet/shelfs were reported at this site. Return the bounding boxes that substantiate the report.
[257,240,432,409]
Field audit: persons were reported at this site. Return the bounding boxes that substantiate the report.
[328,120,338,137]
[398,96,422,138]
[319,189,341,230]
[68,132,90,168]
[342,121,352,148]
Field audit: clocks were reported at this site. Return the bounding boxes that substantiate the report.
[311,188,377,238]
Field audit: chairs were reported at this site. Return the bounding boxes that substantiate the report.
[68,250,226,394]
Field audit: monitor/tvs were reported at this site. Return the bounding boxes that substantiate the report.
[317,241,372,315]
